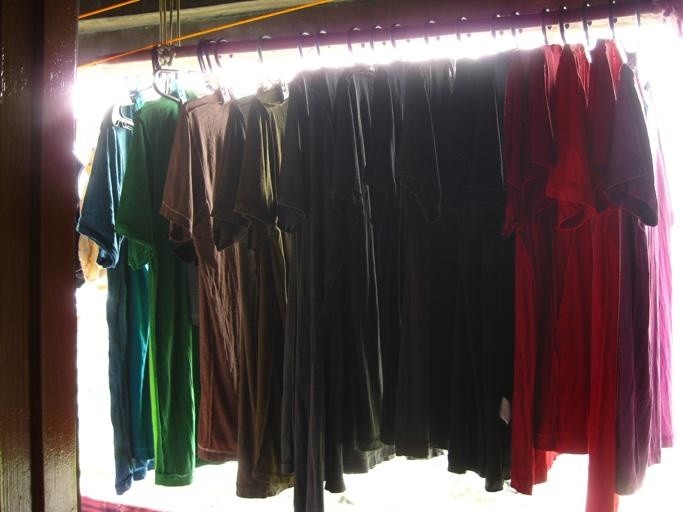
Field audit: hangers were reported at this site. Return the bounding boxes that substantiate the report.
[107,0,682,127]
